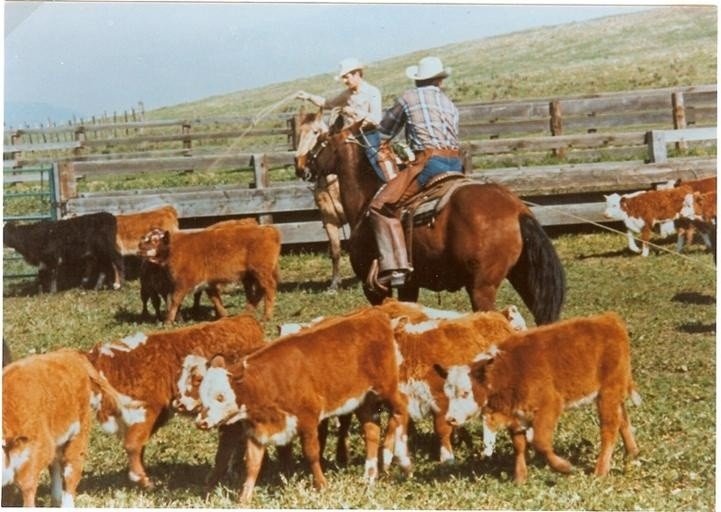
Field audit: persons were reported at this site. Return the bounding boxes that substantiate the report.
[370,57,463,285]
[295,60,389,184]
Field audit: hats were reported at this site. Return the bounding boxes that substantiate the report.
[405,56,454,79]
[335,56,366,82]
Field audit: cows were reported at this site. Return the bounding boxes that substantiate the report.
[3,206,281,328]
[604,177,717,257]
[3,297,640,509]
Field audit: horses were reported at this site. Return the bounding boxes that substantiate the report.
[302,115,565,326]
[291,107,365,297]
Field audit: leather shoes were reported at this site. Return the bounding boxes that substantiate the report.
[373,269,408,287]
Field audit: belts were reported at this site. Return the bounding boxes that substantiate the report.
[424,146,464,158]
